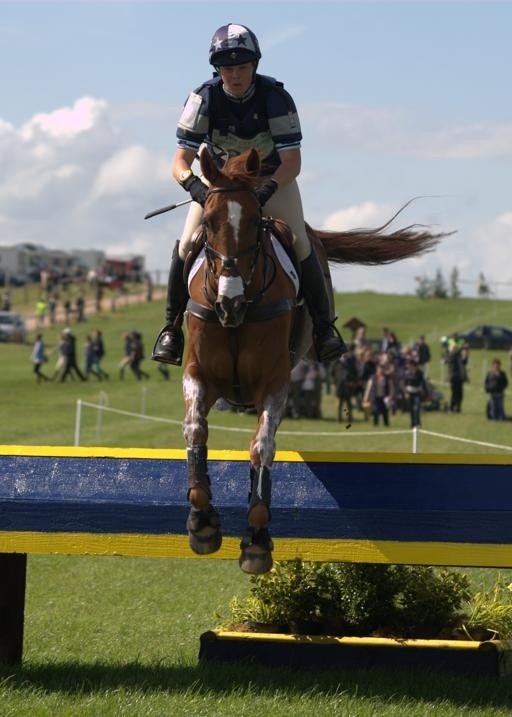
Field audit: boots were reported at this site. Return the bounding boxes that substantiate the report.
[159,240,188,356]
[300,242,343,361]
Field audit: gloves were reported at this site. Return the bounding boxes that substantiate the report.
[182,174,209,207]
[252,179,278,208]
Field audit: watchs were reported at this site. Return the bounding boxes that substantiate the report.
[179,169,194,187]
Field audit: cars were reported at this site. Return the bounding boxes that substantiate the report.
[448,324,512,350]
[0,309,28,343]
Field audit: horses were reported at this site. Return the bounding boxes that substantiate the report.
[181,146,459,575]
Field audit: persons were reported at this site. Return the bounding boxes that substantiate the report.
[0,257,170,383]
[151,23,348,367]
[282,325,512,426]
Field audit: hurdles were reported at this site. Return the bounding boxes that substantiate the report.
[0,445,511,675]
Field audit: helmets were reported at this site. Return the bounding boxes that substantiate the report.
[208,22,262,67]
